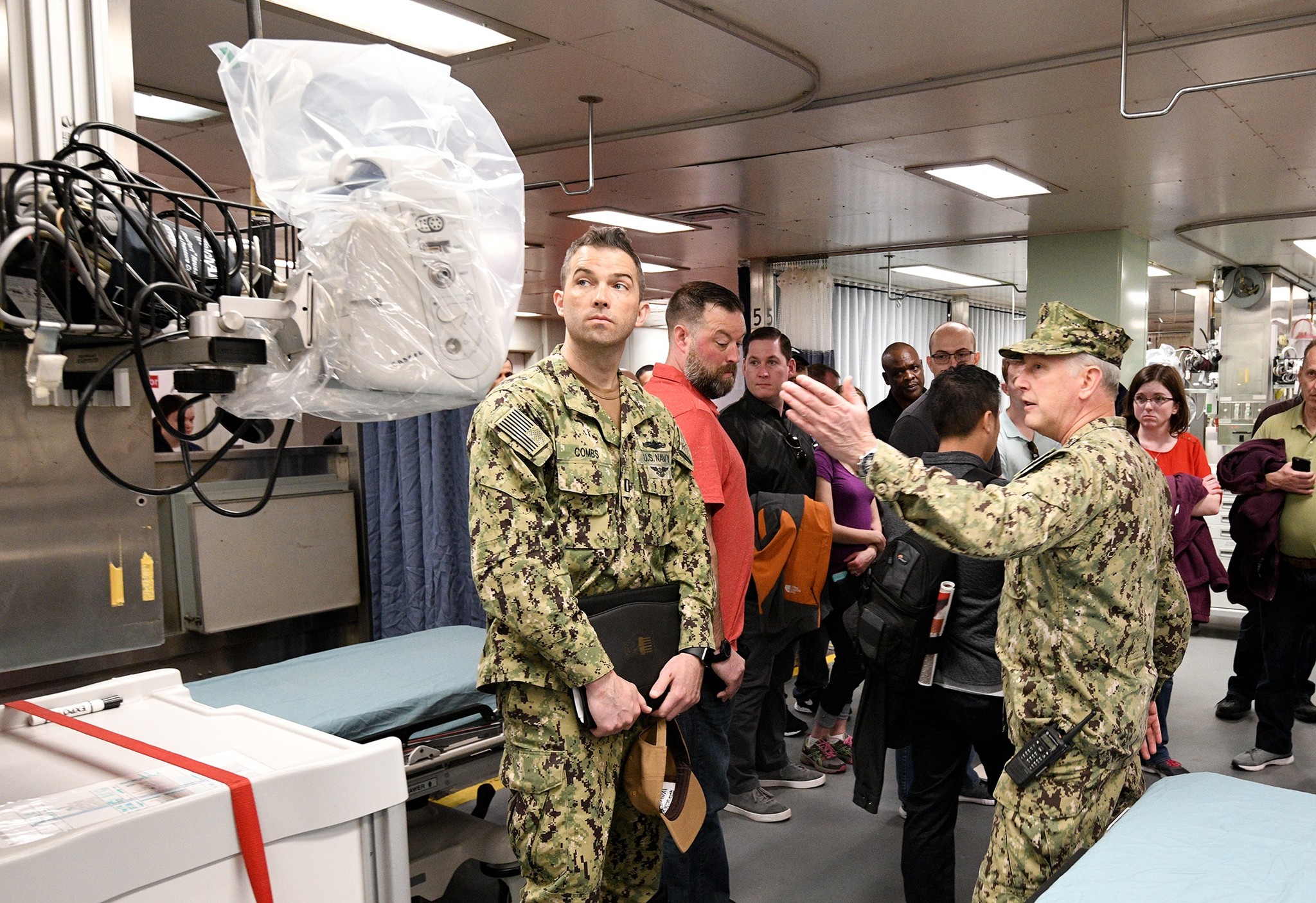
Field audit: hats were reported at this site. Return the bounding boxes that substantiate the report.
[625,717,708,853]
[998,300,1134,370]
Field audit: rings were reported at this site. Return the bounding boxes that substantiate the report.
[854,570,857,574]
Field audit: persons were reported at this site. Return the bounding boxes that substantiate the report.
[882,362,1014,903]
[618,321,1226,822]
[1215,339,1316,770]
[643,281,756,903]
[467,230,714,903]
[779,301,1191,903]
[486,357,513,395]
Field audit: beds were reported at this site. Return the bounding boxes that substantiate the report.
[1032,772,1316,903]
[188,626,503,795]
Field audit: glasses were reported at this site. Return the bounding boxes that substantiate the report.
[932,350,975,364]
[784,434,808,468]
[1132,395,1173,403]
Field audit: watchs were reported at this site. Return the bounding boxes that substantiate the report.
[679,646,715,668]
[857,450,876,477]
[712,638,731,663]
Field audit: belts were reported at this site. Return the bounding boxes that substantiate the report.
[1279,555,1316,570]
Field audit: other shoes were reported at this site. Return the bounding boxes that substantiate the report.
[1215,694,1251,721]
[1293,699,1316,722]
[958,779,994,806]
[898,804,907,819]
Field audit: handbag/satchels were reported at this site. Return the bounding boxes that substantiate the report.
[849,527,955,684]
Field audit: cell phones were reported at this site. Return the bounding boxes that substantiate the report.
[1291,456,1310,472]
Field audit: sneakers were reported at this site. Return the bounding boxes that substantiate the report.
[1141,758,1191,778]
[830,733,853,763]
[1232,746,1294,771]
[725,789,793,823]
[783,704,808,737]
[759,762,826,790]
[800,733,847,774]
[795,693,853,717]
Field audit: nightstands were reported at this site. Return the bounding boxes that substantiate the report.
[0,669,411,903]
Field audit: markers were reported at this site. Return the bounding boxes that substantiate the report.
[26,694,123,726]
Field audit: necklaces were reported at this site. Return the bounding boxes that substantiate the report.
[1139,433,1170,462]
